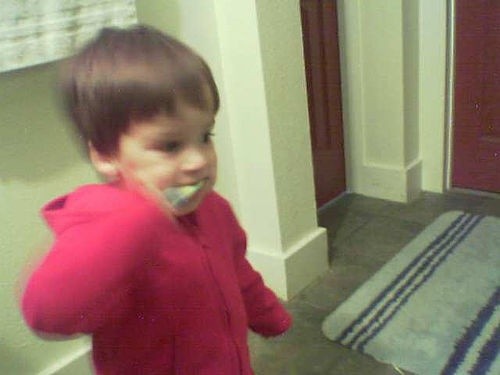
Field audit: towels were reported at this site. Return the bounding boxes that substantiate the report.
[0,0,139,72]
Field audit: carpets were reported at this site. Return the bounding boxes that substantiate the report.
[322,208,500,375]
